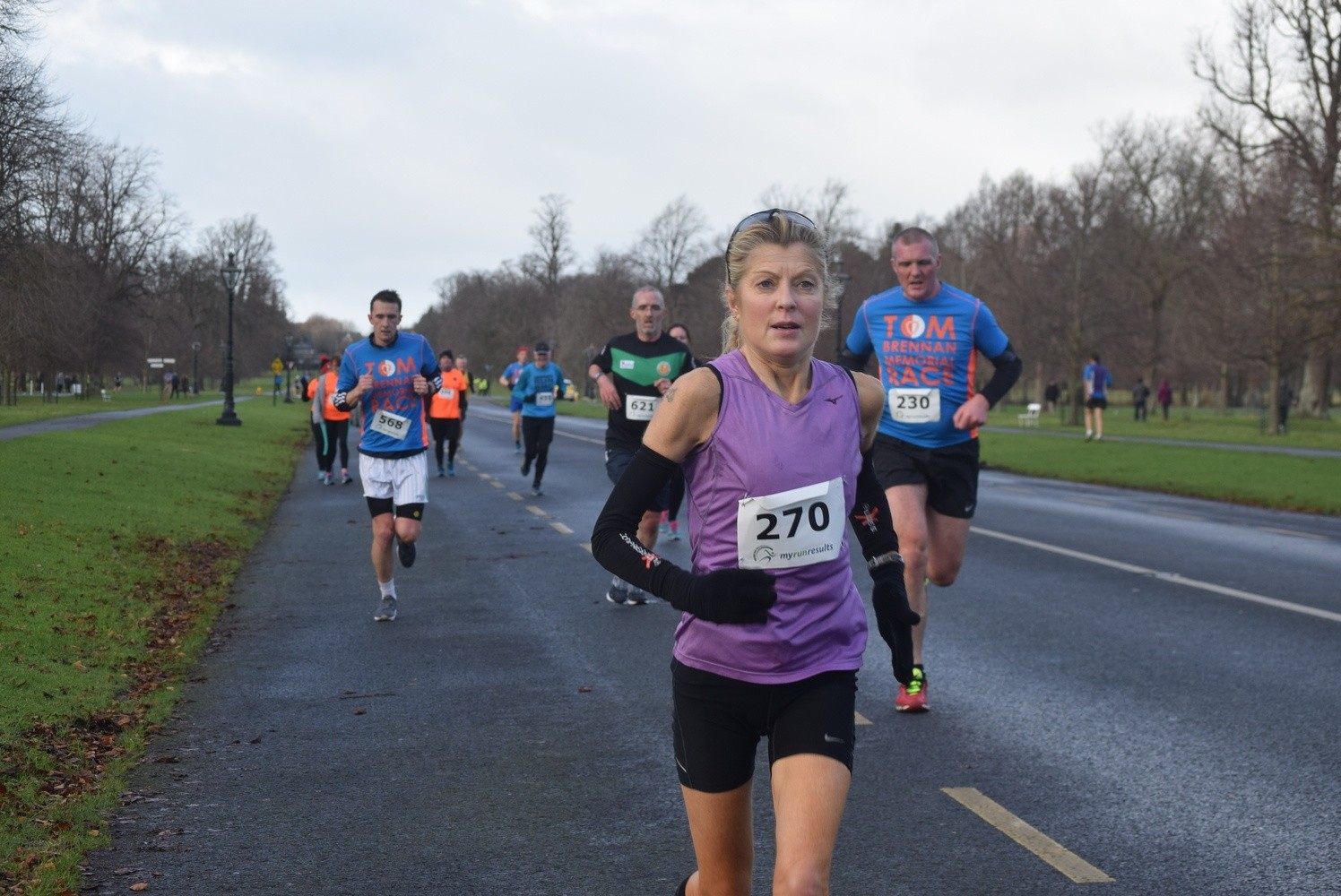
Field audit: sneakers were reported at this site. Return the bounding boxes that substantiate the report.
[374,595,397,621]
[604,575,628,604]
[627,583,648,605]
[892,664,930,710]
[397,538,416,568]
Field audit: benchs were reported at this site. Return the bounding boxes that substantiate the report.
[1018,403,1041,428]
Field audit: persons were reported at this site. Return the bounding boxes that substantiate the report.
[837,225,1020,711]
[33,282,1293,623]
[590,209,922,896]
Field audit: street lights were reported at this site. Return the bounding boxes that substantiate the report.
[186,341,202,394]
[215,251,242,426]
[283,331,296,404]
[829,250,851,365]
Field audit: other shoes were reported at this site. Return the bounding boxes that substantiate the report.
[318,471,326,479]
[341,475,352,485]
[520,457,531,475]
[530,487,543,496]
[323,474,335,485]
[447,464,455,475]
[437,465,445,477]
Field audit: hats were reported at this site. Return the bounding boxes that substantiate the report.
[439,349,453,361]
[535,343,549,355]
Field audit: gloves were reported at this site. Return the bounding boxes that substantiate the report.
[672,569,775,623]
[868,560,922,684]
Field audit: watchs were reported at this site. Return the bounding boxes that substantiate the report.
[425,384,433,395]
[594,371,605,382]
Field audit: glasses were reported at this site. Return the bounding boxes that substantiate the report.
[726,209,819,284]
